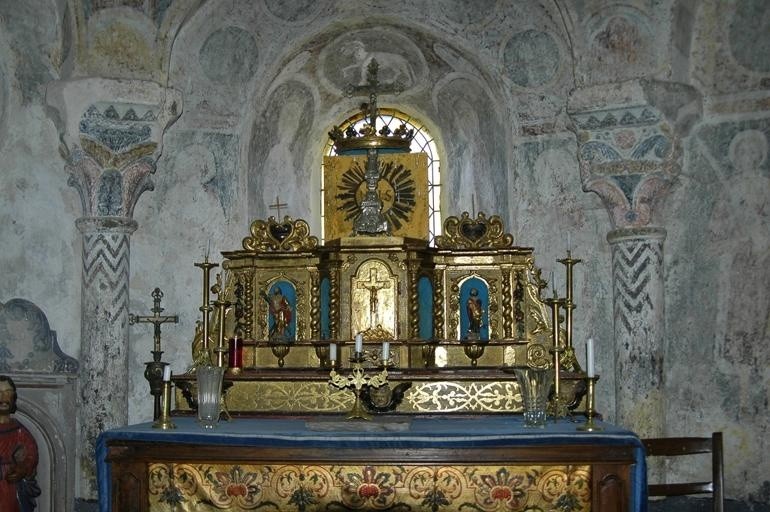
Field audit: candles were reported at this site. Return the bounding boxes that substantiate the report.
[382,342,389,360]
[354,333,363,351]
[329,340,336,361]
[587,337,596,379]
[162,364,172,380]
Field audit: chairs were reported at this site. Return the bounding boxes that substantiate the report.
[640,431,724,512]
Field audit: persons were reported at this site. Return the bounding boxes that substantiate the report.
[0,375,38,512]
[268,285,289,336]
[362,284,387,311]
[467,286,481,324]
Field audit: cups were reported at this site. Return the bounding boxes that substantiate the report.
[512,368,556,429]
[195,365,227,429]
